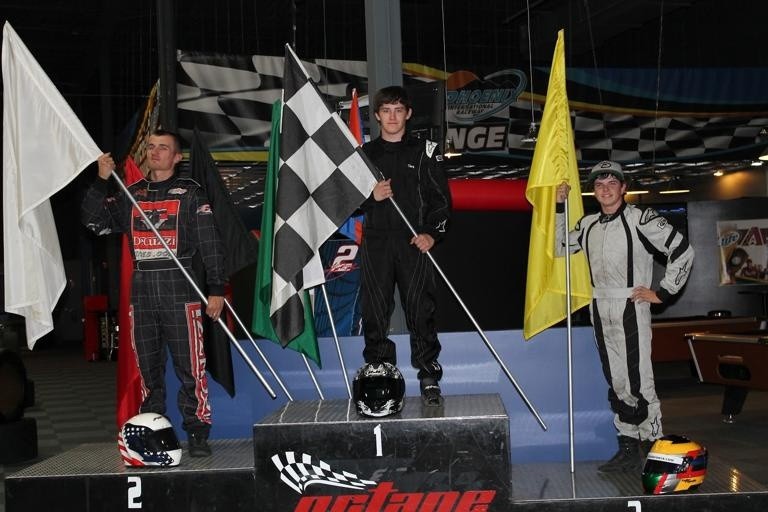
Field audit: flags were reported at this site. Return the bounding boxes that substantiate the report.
[337,84,371,248]
[182,129,258,399]
[520,29,594,339]
[265,41,384,351]
[251,96,323,370]
[0,20,107,350]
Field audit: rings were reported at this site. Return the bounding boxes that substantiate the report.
[213,313,216,317]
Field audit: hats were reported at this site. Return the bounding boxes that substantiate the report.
[588,160,625,184]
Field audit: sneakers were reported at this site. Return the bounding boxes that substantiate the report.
[188,432,211,458]
[420,377,444,408]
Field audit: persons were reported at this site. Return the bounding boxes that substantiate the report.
[357,85,454,407]
[740,259,768,280]
[79,130,228,458]
[554,158,698,475]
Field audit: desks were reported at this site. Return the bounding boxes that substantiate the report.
[686,330,767,424]
[646,313,758,362]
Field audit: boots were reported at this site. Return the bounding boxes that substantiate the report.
[596,435,642,475]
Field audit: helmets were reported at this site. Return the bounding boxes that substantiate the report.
[117,413,183,468]
[352,360,405,417]
[641,433,708,494]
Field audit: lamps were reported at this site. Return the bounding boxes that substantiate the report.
[517,0,545,144]
[431,1,466,160]
[579,173,691,198]
